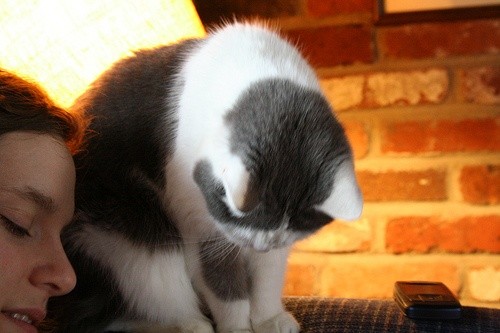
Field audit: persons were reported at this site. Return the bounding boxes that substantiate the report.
[0,69,84,333]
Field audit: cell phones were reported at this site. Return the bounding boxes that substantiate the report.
[393,281,462,320]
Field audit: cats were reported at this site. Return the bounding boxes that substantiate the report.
[66,14,364,333]
